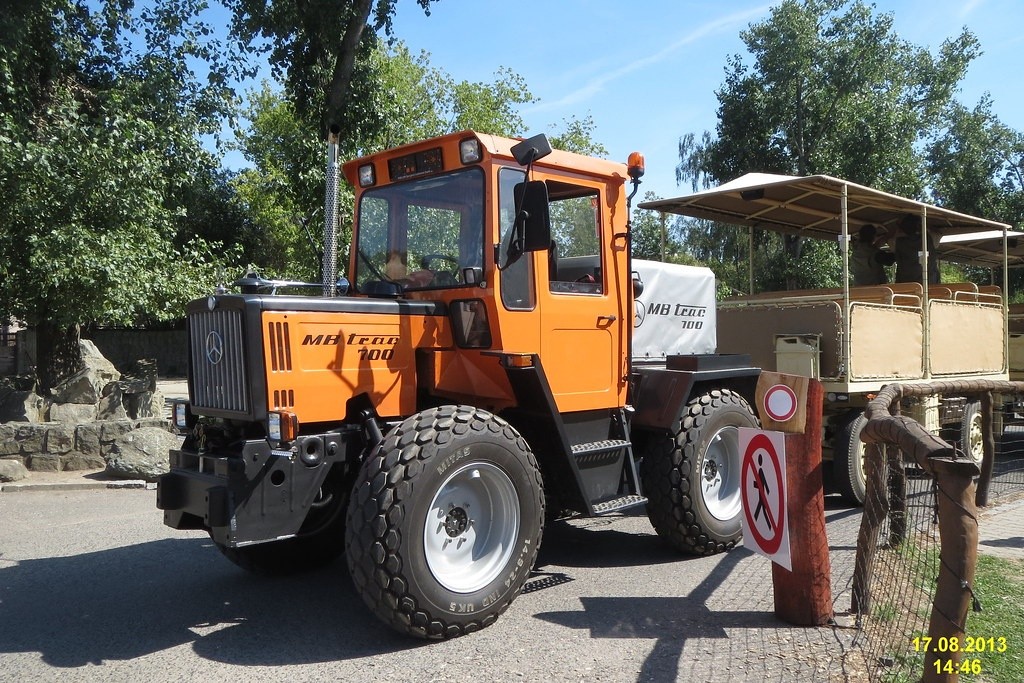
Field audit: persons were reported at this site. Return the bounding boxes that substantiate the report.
[853,224,895,286]
[895,215,940,285]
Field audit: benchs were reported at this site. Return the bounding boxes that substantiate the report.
[723,280,1003,376]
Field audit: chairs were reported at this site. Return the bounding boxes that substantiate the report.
[500,224,528,302]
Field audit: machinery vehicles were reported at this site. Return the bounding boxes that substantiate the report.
[156,121,767,640]
